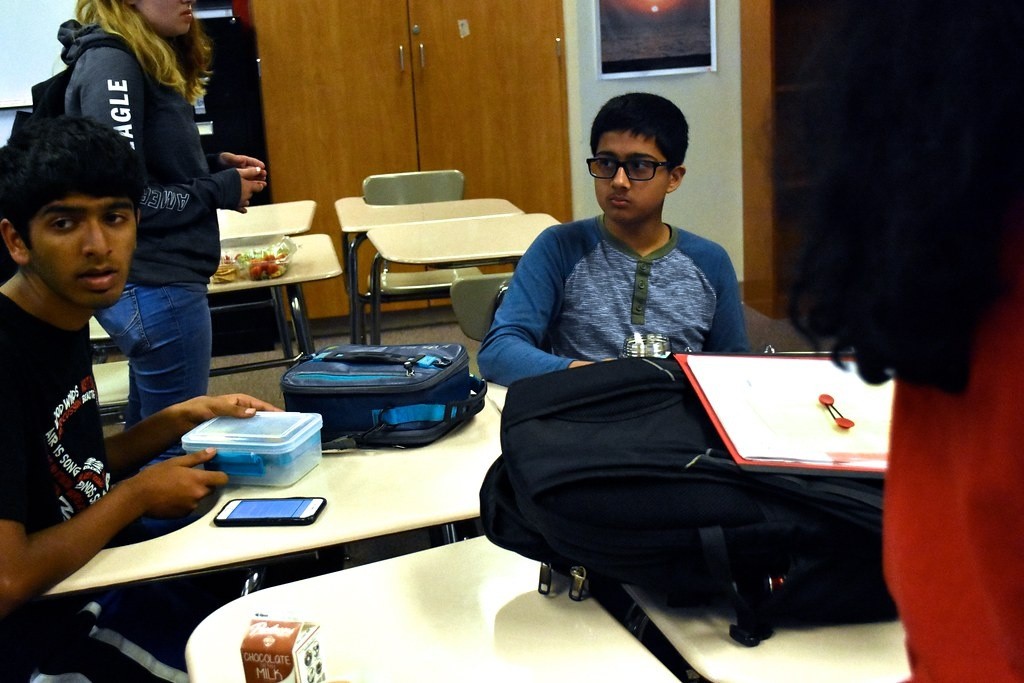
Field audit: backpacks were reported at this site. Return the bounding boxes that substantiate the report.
[478,344,896,647]
[9,40,140,139]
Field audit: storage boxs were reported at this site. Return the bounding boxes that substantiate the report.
[180,410,324,487]
[240,612,327,683]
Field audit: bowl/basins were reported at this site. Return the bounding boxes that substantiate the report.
[212,235,296,284]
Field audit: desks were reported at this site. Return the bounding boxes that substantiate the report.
[335,196,564,353]
[90,199,342,421]
[48,379,916,683]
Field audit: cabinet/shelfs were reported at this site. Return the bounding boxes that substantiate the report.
[250,0,573,339]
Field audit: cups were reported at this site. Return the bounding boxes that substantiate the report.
[624,334,671,360]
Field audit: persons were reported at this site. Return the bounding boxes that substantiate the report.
[56,0,266,461]
[0,116,286,683]
[789,0,1024,683]
[475,92,750,384]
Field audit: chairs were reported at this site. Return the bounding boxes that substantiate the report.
[363,168,516,343]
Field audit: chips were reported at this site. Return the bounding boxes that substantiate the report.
[212,267,239,283]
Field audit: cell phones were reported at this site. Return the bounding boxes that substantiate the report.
[213,495,327,527]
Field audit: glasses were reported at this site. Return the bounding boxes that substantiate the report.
[587,157,671,181]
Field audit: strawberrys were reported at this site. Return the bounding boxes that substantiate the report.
[251,254,286,278]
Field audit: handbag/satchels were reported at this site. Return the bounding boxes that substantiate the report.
[280,342,487,449]
